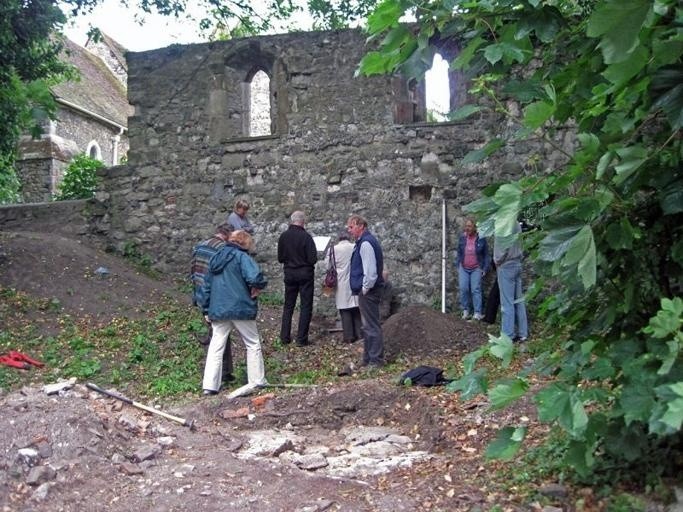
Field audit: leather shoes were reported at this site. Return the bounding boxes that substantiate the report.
[223,373,235,381]
[203,390,216,396]
[280,337,308,347]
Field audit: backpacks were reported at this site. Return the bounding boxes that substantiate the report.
[398,365,452,386]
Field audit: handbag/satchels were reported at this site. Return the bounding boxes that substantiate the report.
[324,269,337,289]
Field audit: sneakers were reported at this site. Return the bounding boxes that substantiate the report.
[462,310,486,320]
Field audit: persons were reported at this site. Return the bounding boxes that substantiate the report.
[324,214,386,372]
[191,199,269,395]
[455,219,491,322]
[493,218,529,344]
[276,210,317,348]
